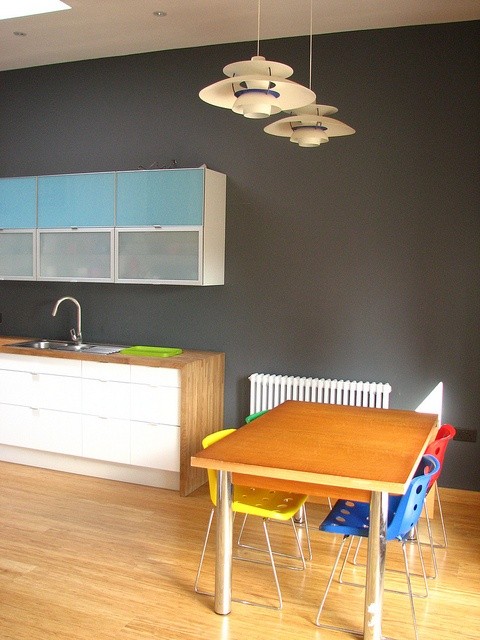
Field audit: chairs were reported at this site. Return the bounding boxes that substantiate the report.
[237,410,337,561]
[353,424,456,580]
[314,454,441,640]
[194,428,309,610]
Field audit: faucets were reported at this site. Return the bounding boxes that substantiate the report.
[51,296,82,342]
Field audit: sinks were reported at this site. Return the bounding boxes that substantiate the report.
[6,339,87,351]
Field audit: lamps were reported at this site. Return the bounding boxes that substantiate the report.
[198,0,316,119]
[263,0,356,148]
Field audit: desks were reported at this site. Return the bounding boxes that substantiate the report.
[190,400,439,640]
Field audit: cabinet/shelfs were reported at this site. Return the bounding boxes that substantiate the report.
[0,164,227,287]
[0,336,226,496]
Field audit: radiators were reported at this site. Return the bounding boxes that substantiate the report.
[248,372,392,414]
